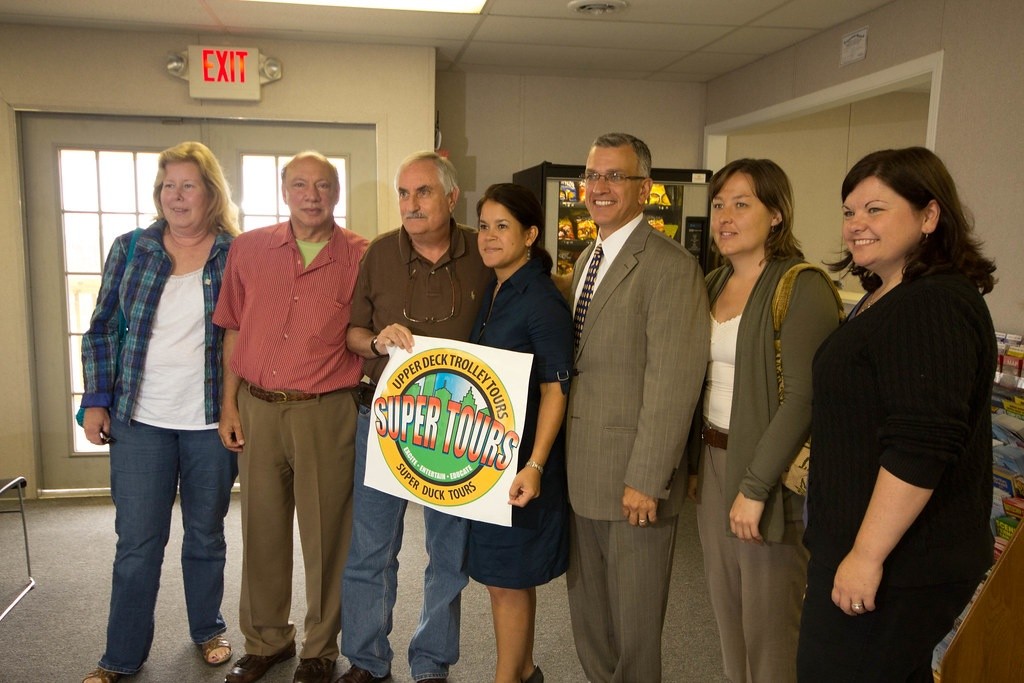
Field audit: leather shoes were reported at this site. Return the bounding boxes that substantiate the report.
[225,640,296,683]
[294,658,334,683]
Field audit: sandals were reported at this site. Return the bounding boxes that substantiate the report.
[82,666,123,683]
[201,635,232,664]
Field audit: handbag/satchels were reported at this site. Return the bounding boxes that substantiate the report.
[771,263,846,496]
[76,228,143,424]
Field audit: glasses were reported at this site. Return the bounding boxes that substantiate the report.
[580,174,645,184]
[404,266,455,323]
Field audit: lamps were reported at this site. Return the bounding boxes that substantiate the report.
[166,43,281,100]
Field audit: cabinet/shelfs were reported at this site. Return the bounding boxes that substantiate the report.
[512,160,713,277]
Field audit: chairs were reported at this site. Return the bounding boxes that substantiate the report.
[0,477,35,622]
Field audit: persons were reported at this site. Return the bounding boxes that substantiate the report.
[797,146,999,683]
[329,150,495,683]
[81,140,242,683]
[211,150,372,682]
[566,129,712,683]
[688,156,844,683]
[467,182,573,683]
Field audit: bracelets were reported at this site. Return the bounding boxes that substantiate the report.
[527,462,544,475]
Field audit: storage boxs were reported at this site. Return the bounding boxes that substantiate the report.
[932,327,1024,683]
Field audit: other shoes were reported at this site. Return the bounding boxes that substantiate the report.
[418,678,448,683]
[335,665,391,683]
[522,664,545,683]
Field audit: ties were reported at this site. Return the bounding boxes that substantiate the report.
[573,244,603,354]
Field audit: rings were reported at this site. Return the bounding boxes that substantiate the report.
[851,602,863,610]
[639,519,648,523]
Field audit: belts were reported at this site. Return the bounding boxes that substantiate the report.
[242,379,335,403]
[700,425,727,449]
